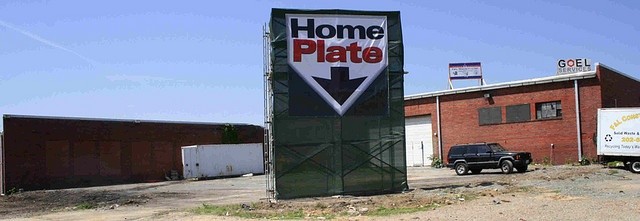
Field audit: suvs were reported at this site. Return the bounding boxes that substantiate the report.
[446,142,532,176]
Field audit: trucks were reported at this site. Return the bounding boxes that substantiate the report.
[596,106,640,174]
